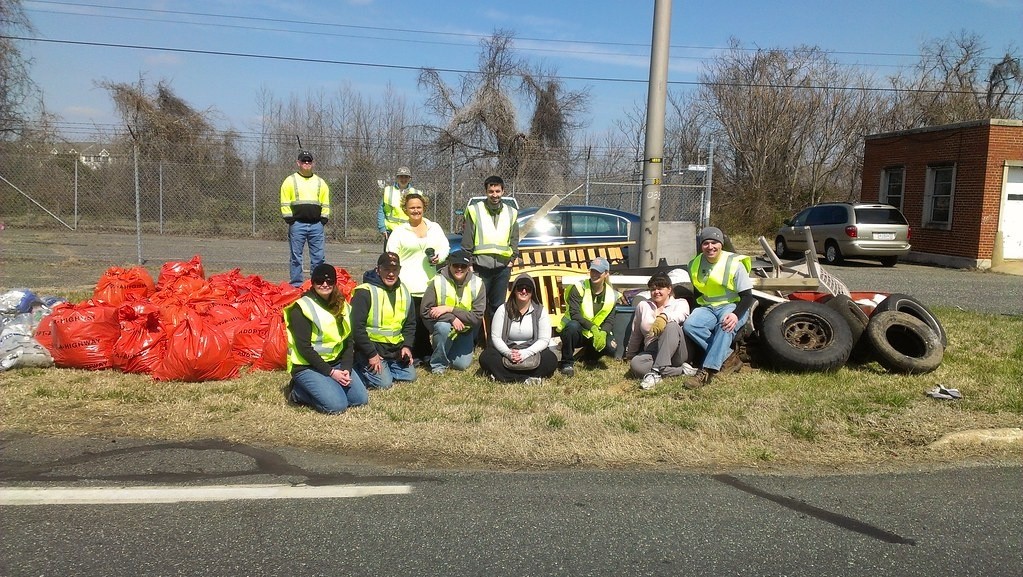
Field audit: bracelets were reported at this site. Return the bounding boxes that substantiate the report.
[330,369,335,378]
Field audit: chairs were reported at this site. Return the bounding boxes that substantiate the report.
[755,225,851,299]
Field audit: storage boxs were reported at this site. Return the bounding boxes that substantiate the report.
[614,307,638,360]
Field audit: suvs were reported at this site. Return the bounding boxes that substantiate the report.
[774,200,912,267]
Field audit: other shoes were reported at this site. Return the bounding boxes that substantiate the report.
[413,358,423,368]
[432,365,444,377]
[487,371,495,381]
[524,376,541,386]
[424,355,431,365]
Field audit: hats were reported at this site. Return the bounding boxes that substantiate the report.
[298,151,313,161]
[378,252,400,267]
[397,166,411,178]
[515,278,534,289]
[699,227,724,246]
[450,250,470,266]
[312,264,337,282]
[400,188,429,214]
[588,257,609,273]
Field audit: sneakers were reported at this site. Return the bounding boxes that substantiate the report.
[684,365,712,390]
[640,372,663,390]
[720,352,744,375]
[289,379,305,406]
[681,363,698,375]
[561,365,574,377]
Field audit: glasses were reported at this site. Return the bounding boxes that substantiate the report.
[314,274,335,286]
[516,285,532,294]
[301,157,312,162]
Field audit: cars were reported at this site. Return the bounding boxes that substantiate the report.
[436,204,736,273]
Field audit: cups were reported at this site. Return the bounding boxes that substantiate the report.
[425,248,435,266]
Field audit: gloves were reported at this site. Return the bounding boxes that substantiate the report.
[649,315,668,338]
[591,325,607,352]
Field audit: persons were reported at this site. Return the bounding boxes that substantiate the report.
[350,252,417,389]
[282,264,368,414]
[479,274,558,386]
[555,257,622,376]
[377,167,427,252]
[420,250,487,375]
[386,194,450,368]
[462,176,520,348]
[280,151,330,289]
[626,272,699,389]
[683,227,752,389]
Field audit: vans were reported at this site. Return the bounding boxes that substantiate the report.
[453,195,520,231]
